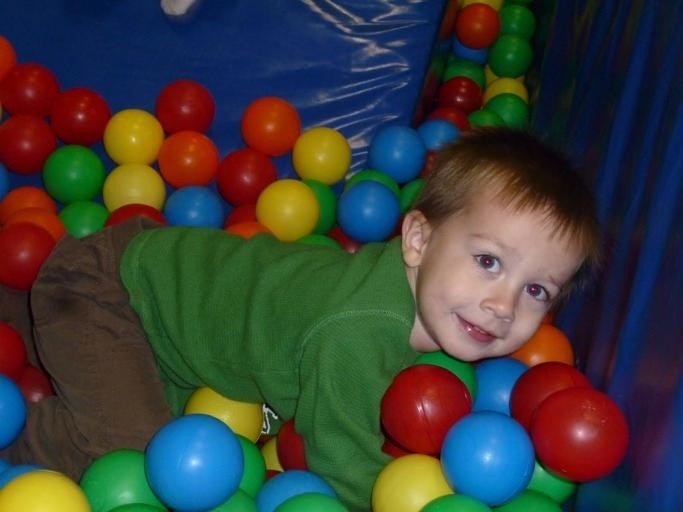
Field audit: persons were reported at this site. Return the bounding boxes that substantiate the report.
[2,124,612,511]
